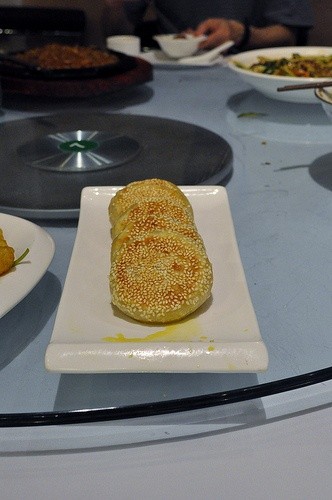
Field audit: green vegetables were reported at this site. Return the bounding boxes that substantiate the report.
[233,53,332,118]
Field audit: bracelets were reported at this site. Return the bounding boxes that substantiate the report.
[240,19,250,52]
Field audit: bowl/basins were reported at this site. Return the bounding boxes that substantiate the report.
[228,46,332,104]
[153,33,207,58]
[314,87,332,117]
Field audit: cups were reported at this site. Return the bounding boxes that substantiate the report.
[107,35,140,58]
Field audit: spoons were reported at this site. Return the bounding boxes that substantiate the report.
[180,40,234,63]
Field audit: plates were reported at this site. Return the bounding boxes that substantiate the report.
[0,212,55,318]
[45,185,269,374]
[141,50,223,67]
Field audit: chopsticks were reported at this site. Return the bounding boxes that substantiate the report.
[277,81,332,92]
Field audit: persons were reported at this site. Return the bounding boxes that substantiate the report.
[103,0,315,57]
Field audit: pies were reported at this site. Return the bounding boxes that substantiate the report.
[108,179,213,323]
